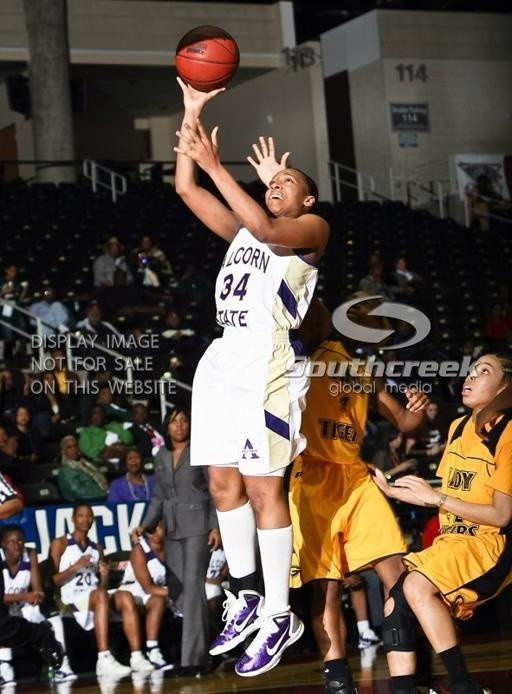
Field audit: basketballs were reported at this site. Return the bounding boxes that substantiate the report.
[176,24,237,91]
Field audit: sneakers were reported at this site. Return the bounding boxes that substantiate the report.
[208,589,305,677]
[96,645,174,675]
[358,629,382,648]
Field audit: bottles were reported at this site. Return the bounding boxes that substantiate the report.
[47,665,56,689]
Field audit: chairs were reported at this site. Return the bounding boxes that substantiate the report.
[0,171,511,672]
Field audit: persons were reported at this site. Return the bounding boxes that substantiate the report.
[0,76,512,694]
